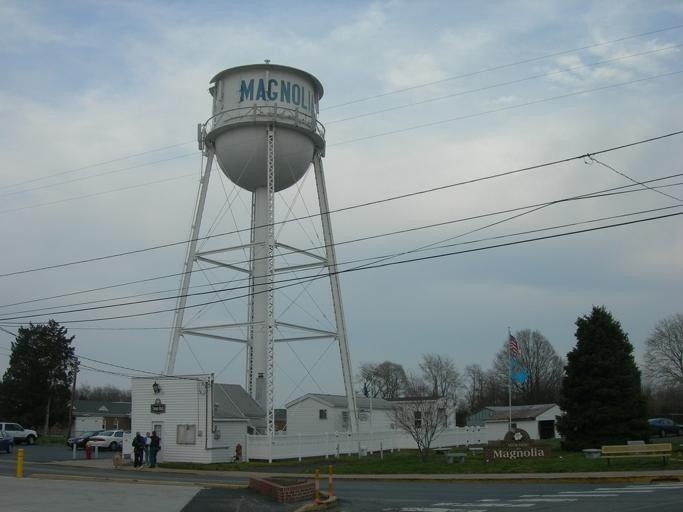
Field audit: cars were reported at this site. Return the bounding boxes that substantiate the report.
[67,430,123,450]
[0,422,38,454]
[648,418,683,437]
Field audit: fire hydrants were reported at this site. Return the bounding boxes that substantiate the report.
[84,445,93,458]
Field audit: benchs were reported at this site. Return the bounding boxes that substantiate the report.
[581,441,672,468]
[432,447,484,464]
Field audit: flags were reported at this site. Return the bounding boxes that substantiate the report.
[510,359,529,384]
[509,333,519,361]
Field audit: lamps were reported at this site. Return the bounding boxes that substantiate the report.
[152,381,160,394]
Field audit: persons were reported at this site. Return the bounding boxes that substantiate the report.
[144,431,152,466]
[131,431,145,467]
[84,439,94,459]
[148,430,160,467]
